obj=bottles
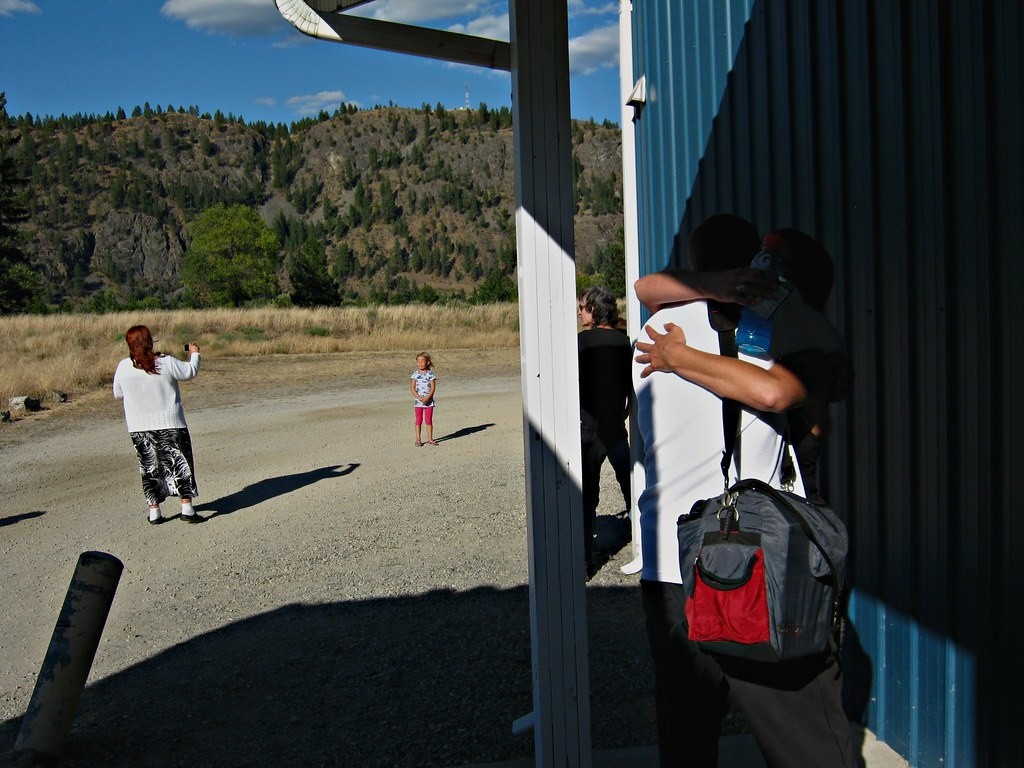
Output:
[734,232,790,357]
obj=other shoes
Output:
[428,441,439,446]
[147,515,166,524]
[180,511,204,523]
[415,440,422,446]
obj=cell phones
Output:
[185,344,189,351]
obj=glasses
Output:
[579,304,585,312]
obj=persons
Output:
[112,324,204,524]
[578,285,632,579]
[410,352,440,446]
[629,214,854,767]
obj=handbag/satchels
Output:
[676,478,850,664]
